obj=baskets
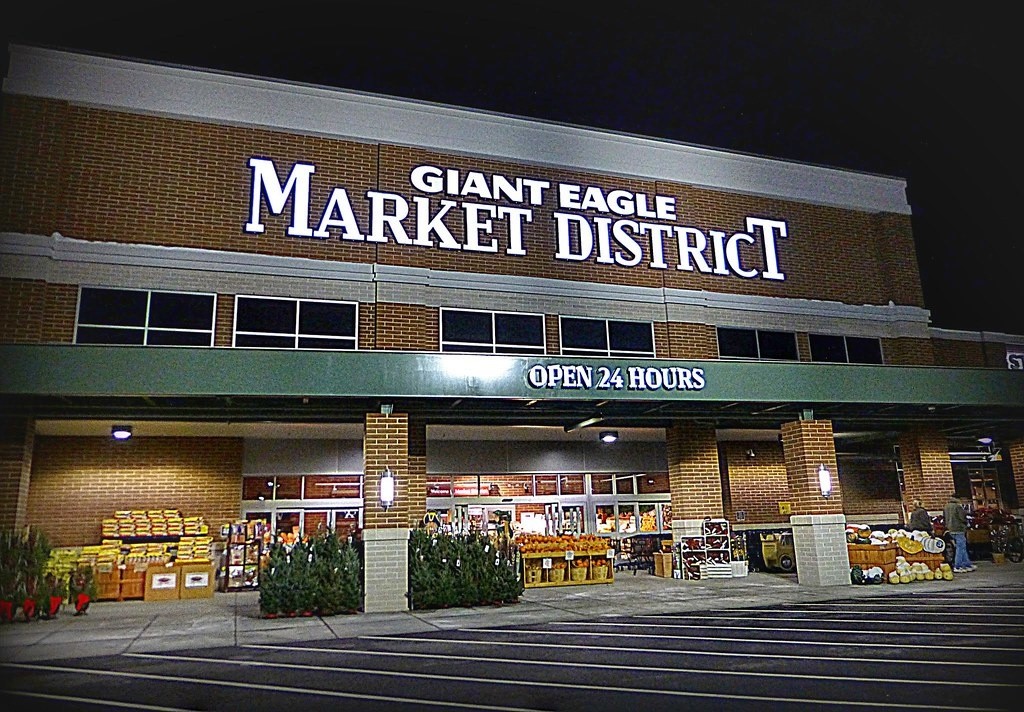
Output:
[549,569,564,582]
[592,561,610,580]
[571,567,587,581]
[526,569,542,582]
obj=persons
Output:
[944,493,978,572]
[908,499,936,538]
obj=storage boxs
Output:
[729,561,749,577]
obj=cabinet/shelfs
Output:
[673,520,732,580]
[71,522,261,604]
[520,548,614,589]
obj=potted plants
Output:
[409,529,525,610]
[258,529,366,619]
[0,527,98,623]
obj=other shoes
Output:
[954,567,967,573]
[966,566,977,571]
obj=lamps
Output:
[818,463,831,499]
[381,468,395,509]
[599,431,618,443]
[111,426,132,439]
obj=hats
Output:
[952,493,960,498]
